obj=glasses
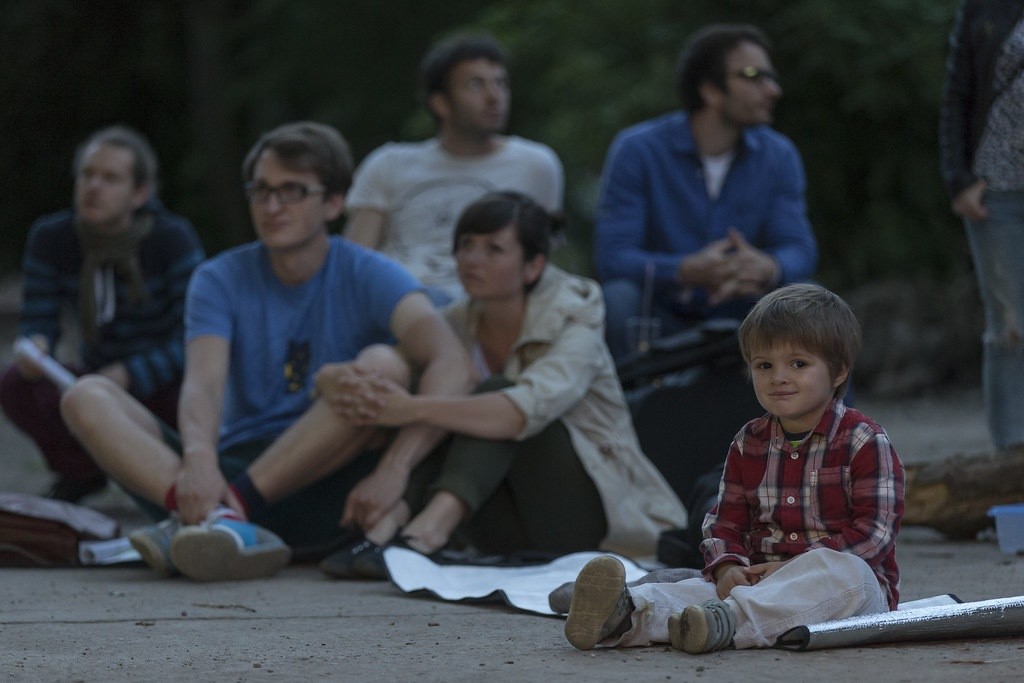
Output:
[727,65,781,85]
[241,181,328,205]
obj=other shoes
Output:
[41,470,109,504]
[667,598,738,654]
[320,535,381,577]
[356,524,450,581]
[563,555,632,651]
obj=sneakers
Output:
[168,515,291,583]
[128,508,186,574]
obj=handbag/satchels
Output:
[616,259,772,478]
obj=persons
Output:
[0,24,820,582]
[564,283,904,654]
[941,0,1024,452]
